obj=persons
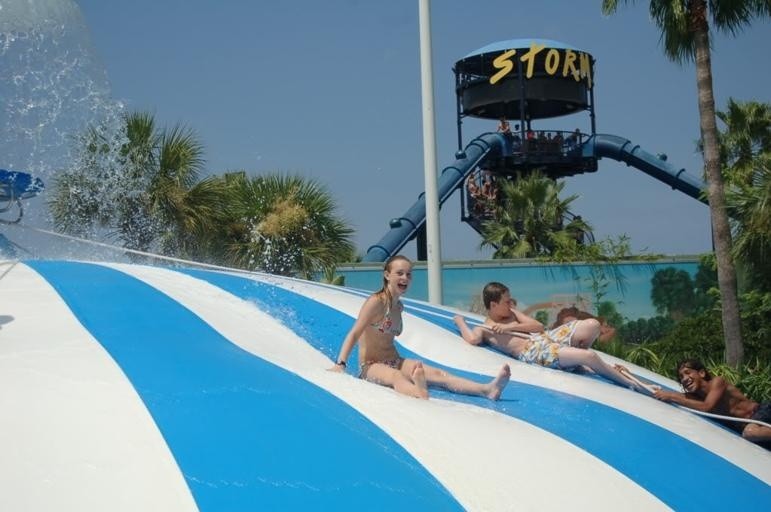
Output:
[571,129,580,144]
[552,132,564,144]
[335,360,348,368]
[495,118,511,139]
[324,254,513,401]
[512,124,522,140]
[453,281,662,400]
[537,131,547,141]
[468,177,500,218]
[651,358,771,443]
